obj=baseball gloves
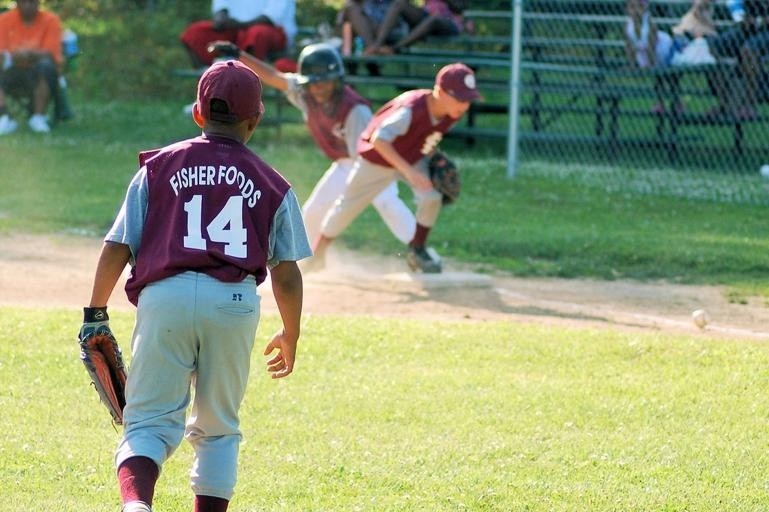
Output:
[79,306,126,424]
[429,154,460,204]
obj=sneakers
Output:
[123,502,150,512]
[0,114,51,134]
[404,241,441,274]
[298,253,327,274]
[651,101,756,119]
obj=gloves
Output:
[208,41,242,60]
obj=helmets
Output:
[297,44,345,85]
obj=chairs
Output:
[0,41,72,126]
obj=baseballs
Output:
[692,310,707,325]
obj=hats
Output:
[197,61,265,124]
[436,64,483,102]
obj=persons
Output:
[207,39,443,271]
[304,62,485,274]
[0,0,64,135]
[181,0,299,115]
[624,0,769,118]
[79,59,313,512]
[339,0,467,77]
